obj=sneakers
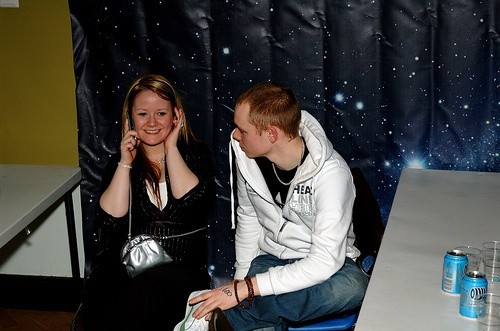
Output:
[173,291,209,331]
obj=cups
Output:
[453,240,500,331]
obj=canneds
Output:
[459,270,488,320]
[441,250,468,296]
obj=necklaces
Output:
[272,137,304,186]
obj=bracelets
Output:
[118,161,133,169]
[233,276,254,310]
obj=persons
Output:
[173,82,365,331]
[72,73,219,331]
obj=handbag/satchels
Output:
[121,235,174,287]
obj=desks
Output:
[353,168,500,331]
[0,163,81,312]
